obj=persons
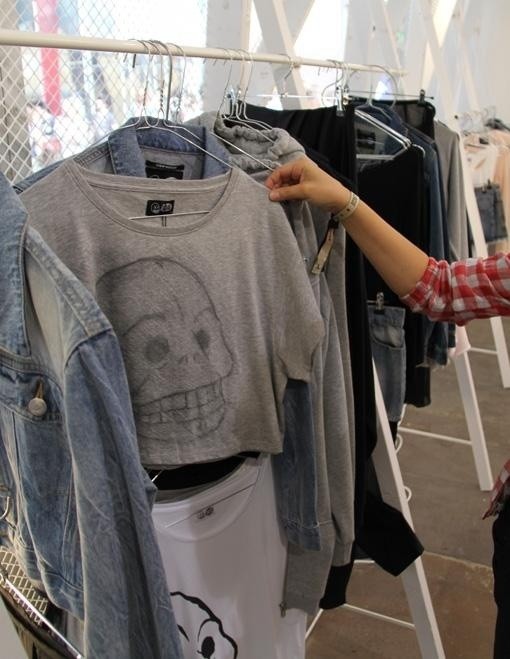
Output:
[265,153,510,658]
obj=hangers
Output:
[9,38,304,225]
[243,51,435,163]
[458,103,510,158]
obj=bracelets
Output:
[332,191,360,221]
